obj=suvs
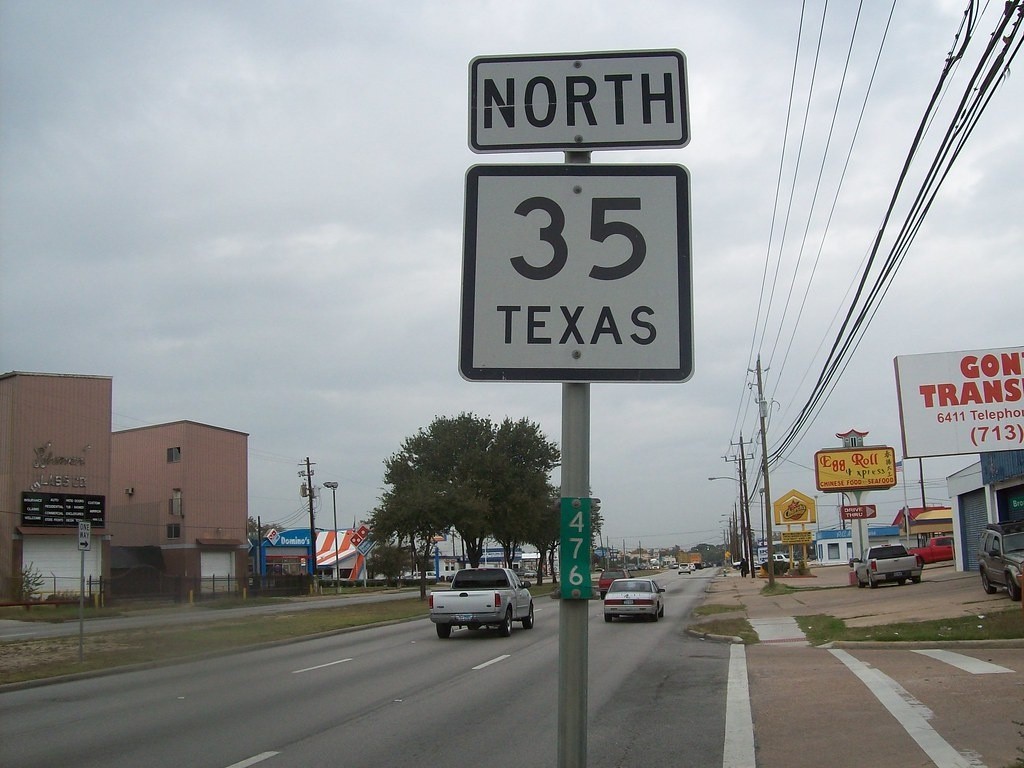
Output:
[977,519,1024,601]
[598,567,635,600]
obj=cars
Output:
[732,551,801,571]
[668,562,679,569]
[677,562,691,574]
[603,578,666,621]
[689,563,696,571]
[694,562,713,569]
[524,569,538,578]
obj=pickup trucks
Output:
[852,544,925,590]
[906,536,955,568]
[428,567,534,639]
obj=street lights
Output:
[708,476,756,578]
[323,481,341,594]
